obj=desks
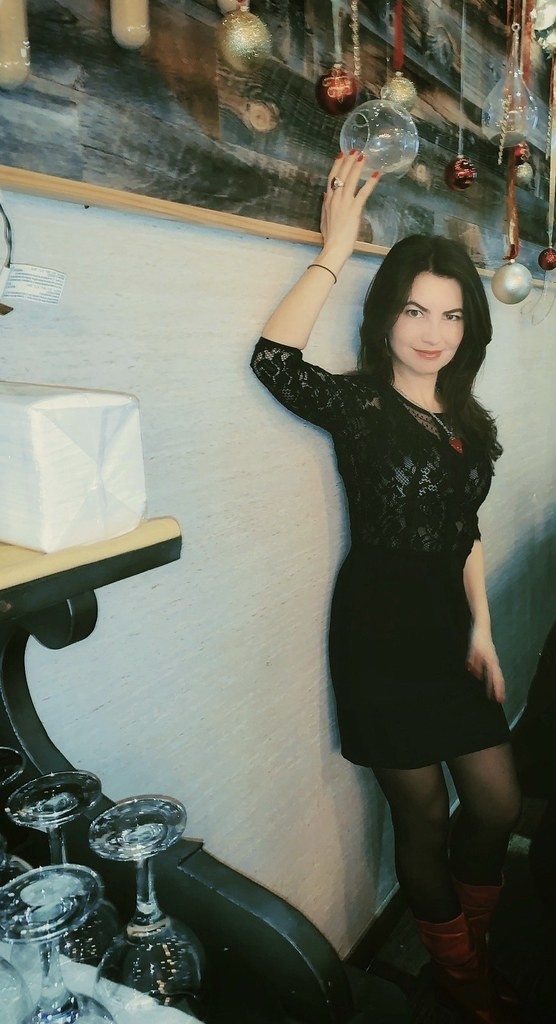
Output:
[0,517,357,1024]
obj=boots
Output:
[450,871,525,1008]
[414,912,527,1024]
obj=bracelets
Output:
[307,264,337,284]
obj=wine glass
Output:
[0,745,208,1023]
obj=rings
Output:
[330,177,344,189]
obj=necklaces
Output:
[394,384,463,453]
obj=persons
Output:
[250,151,520,1024]
